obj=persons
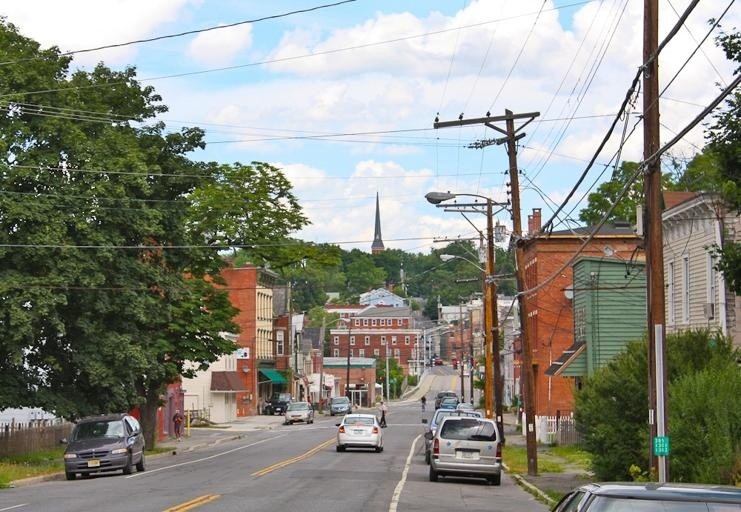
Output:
[173,409,183,441]
[421,396,426,412]
[468,357,474,369]
[379,401,387,427]
[318,397,324,411]
[308,395,312,405]
[452,360,457,370]
[300,385,305,402]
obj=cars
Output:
[61,413,147,479]
[331,397,351,416]
[336,414,384,453]
[286,402,314,424]
[425,408,502,485]
[548,480,741,512]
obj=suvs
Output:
[267,392,292,414]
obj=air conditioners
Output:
[703,303,714,319]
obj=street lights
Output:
[425,191,508,438]
[319,317,351,413]
